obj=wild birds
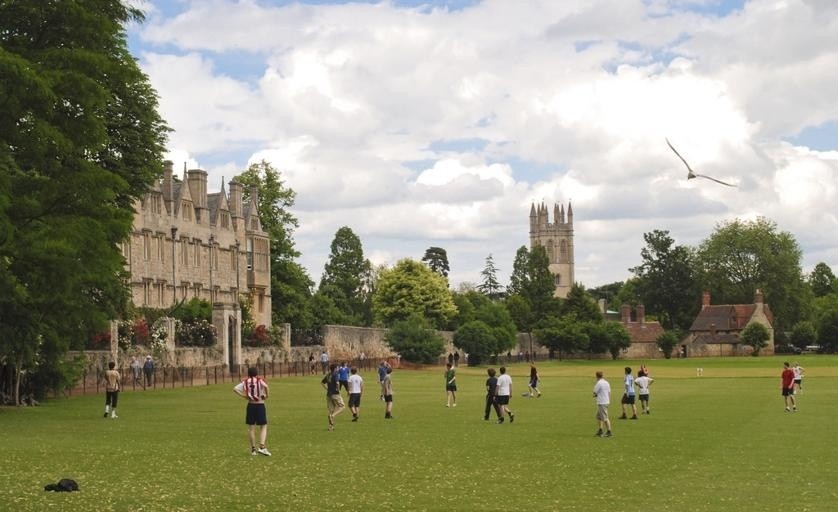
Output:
[663,135,739,190]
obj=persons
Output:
[483,348,541,423]
[792,362,806,395]
[309,349,392,431]
[618,367,637,419]
[780,362,796,411]
[104,362,120,419]
[143,355,155,386]
[593,371,612,437]
[635,371,655,414]
[233,367,271,456]
[129,357,142,390]
[444,347,460,408]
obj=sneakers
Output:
[530,392,542,399]
[618,409,650,420]
[793,391,797,395]
[785,404,797,412]
[251,448,257,456]
[103,410,109,417]
[351,413,359,422]
[110,416,118,419]
[480,412,514,424]
[257,447,272,456]
[385,415,392,419]
[592,431,612,437]
[327,414,335,432]
[446,402,456,407]
